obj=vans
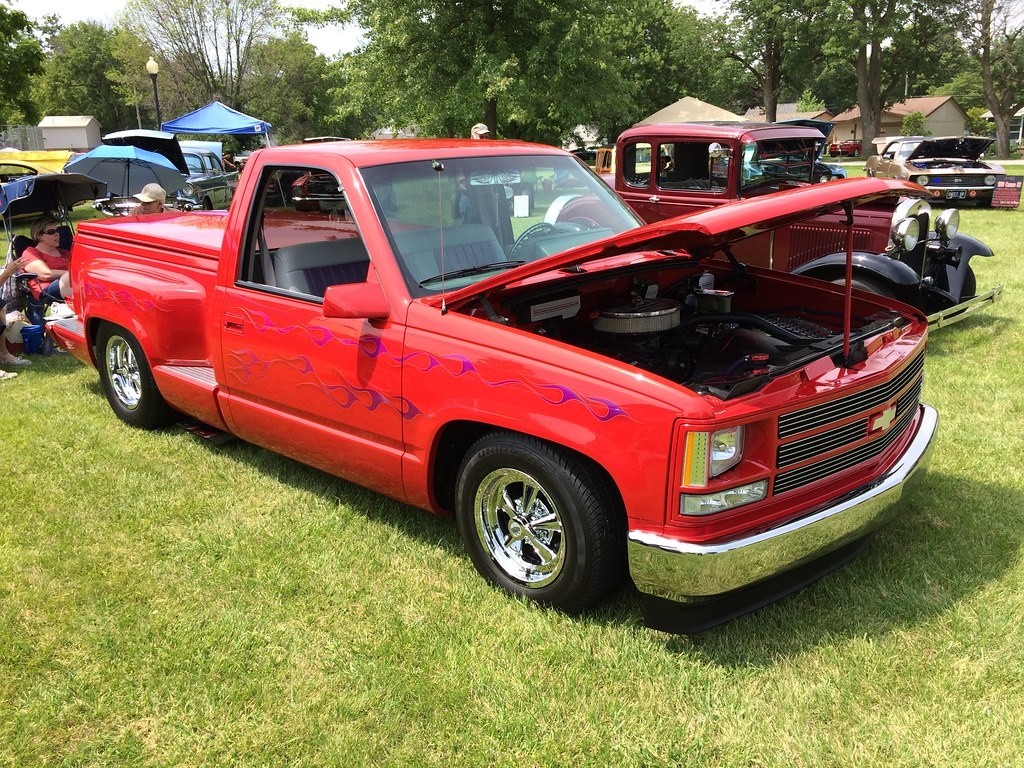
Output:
[595,140,673,174]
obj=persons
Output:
[128,182,182,215]
[221,150,236,172]
[18,216,73,302]
[0,256,32,380]
[456,123,515,248]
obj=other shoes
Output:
[0,370,18,379]
[1,356,32,365]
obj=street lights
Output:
[146,56,161,130]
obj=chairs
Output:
[14,226,75,333]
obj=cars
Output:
[100,130,238,212]
[829,139,862,156]
[47,138,939,611]
[862,133,1005,205]
[291,135,350,212]
[544,125,1003,332]
[711,119,847,191]
[0,160,88,222]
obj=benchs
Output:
[275,222,508,300]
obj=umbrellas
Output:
[63,145,187,198]
[628,94,752,127]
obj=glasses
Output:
[480,136,489,139]
[43,228,60,235]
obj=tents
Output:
[160,101,286,209]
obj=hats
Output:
[133,183,166,203]
[471,123,490,135]
